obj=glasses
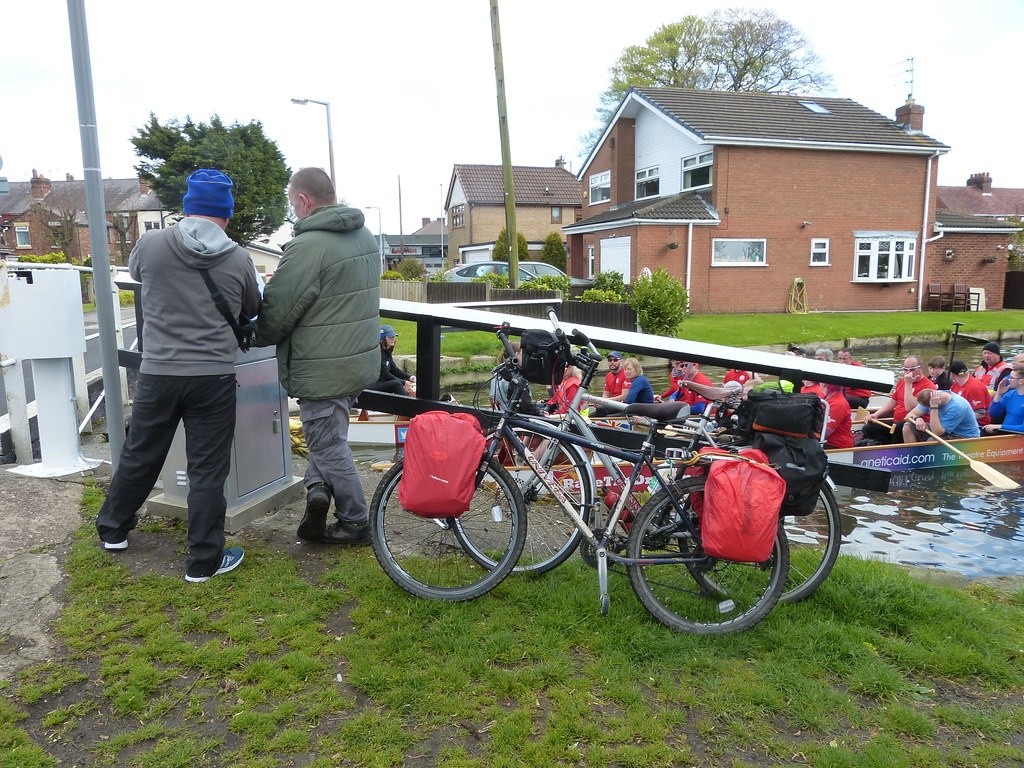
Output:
[608,359,620,362]
[1008,377,1022,382]
[680,364,688,368]
[902,366,921,372]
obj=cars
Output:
[483,260,596,302]
[441,261,541,286]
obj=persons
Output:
[653,359,714,415]
[490,341,543,415]
[1002,353,1024,396]
[980,365,1024,437]
[781,346,871,450]
[95,169,262,582]
[947,361,991,428]
[544,365,589,416]
[589,350,631,418]
[903,388,980,444]
[715,368,764,446]
[249,169,381,548]
[623,358,654,403]
[972,342,1013,400]
[373,324,416,397]
[926,356,949,391]
[862,356,936,442]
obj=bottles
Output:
[704,420,719,431]
[647,458,677,494]
[602,485,636,531]
[580,400,590,418]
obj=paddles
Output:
[853,406,895,433]
[904,416,1020,489]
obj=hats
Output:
[949,361,968,375]
[182,169,234,218]
[380,325,399,340]
[607,351,622,360]
[789,346,806,354]
[982,342,1000,355]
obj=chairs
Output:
[927,283,955,310]
[952,283,981,312]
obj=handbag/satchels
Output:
[519,329,565,386]
[690,446,769,514]
[753,432,829,516]
[237,312,259,353]
[740,389,823,441]
[701,458,786,563]
[398,411,486,518]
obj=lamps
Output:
[800,220,811,230]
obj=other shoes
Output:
[319,510,373,545]
[297,486,331,540]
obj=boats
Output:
[284,385,1023,495]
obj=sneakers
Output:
[185,547,244,582]
[102,535,129,549]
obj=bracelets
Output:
[930,407,938,410]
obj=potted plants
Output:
[984,256,998,263]
[667,241,680,249]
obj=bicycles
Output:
[364,319,792,636]
[444,302,845,609]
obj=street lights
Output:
[365,205,384,274]
[290,97,336,194]
